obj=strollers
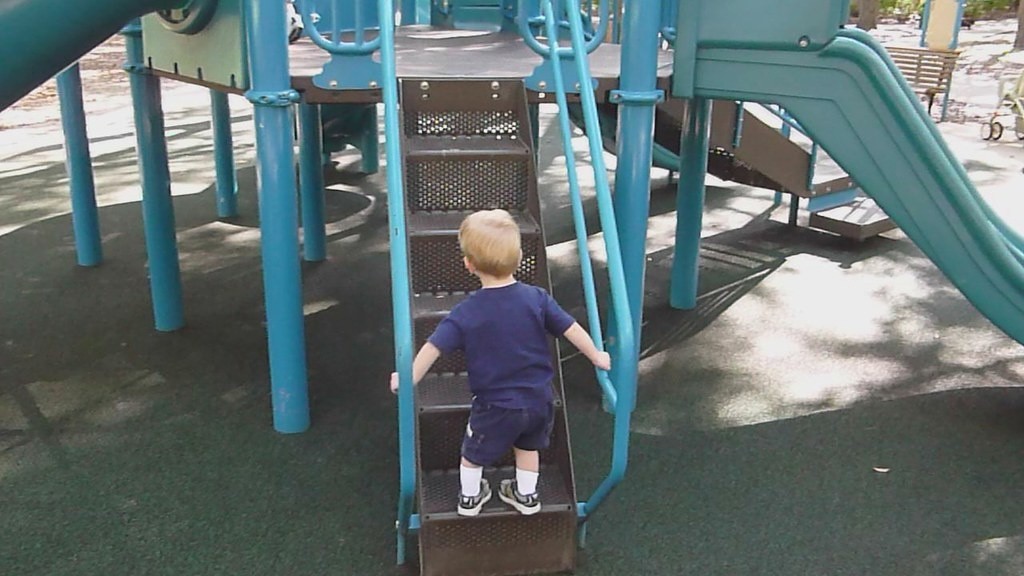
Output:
[979,51,1024,142]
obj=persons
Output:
[390,209,611,517]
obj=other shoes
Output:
[288,14,304,43]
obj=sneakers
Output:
[496,478,542,516]
[456,479,492,516]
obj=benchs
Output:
[883,46,960,122]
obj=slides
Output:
[563,97,682,169]
[800,25,1024,347]
